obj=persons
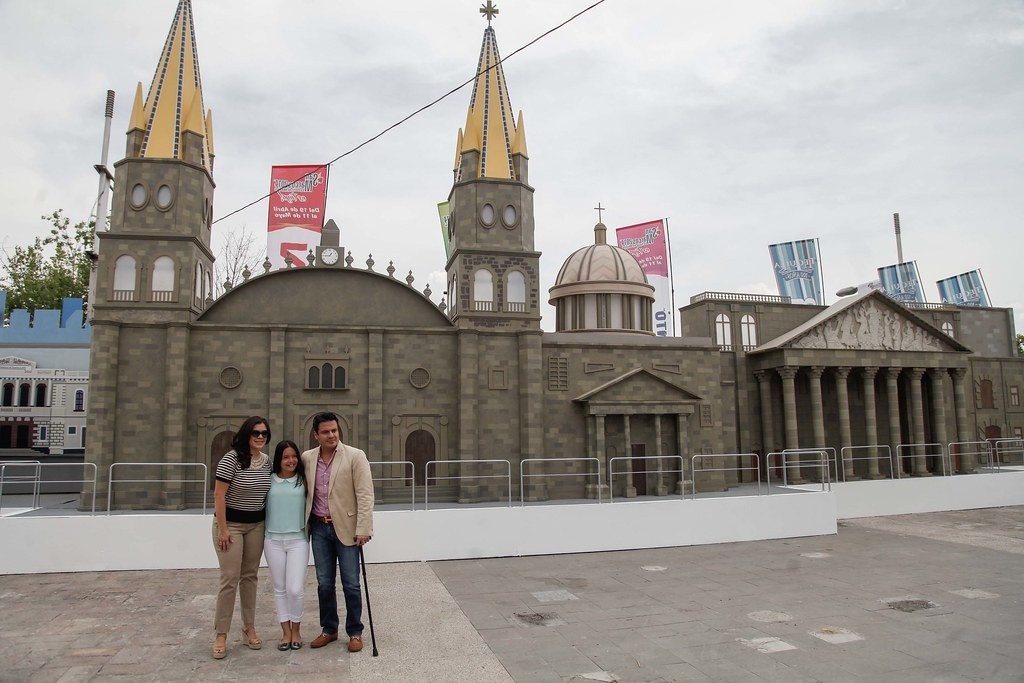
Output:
[794,299,942,351]
[264,440,310,649]
[211,416,273,659]
[300,413,375,652]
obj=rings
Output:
[361,541,365,544]
[219,544,222,545]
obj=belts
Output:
[313,515,332,523]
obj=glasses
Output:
[251,430,270,439]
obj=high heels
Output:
[241,625,261,649]
[213,633,227,658]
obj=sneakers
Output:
[311,633,338,647]
[348,636,362,651]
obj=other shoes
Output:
[278,639,291,650]
[291,641,302,649]
